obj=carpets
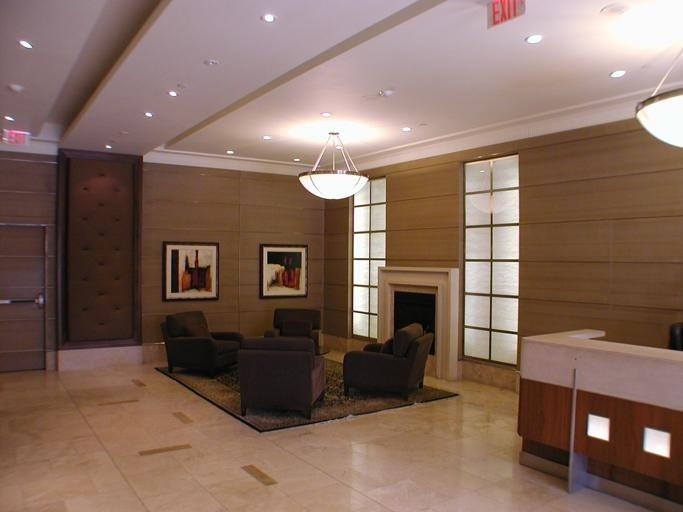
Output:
[154,353,459,433]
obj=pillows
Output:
[192,327,215,344]
[282,327,310,336]
[379,337,392,353]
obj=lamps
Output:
[297,131,369,200]
[631,48,683,148]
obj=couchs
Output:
[159,310,245,381]
[263,307,323,354]
[342,323,436,399]
[237,336,324,420]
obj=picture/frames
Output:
[160,240,220,301]
[258,243,308,299]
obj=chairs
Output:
[667,323,682,351]
